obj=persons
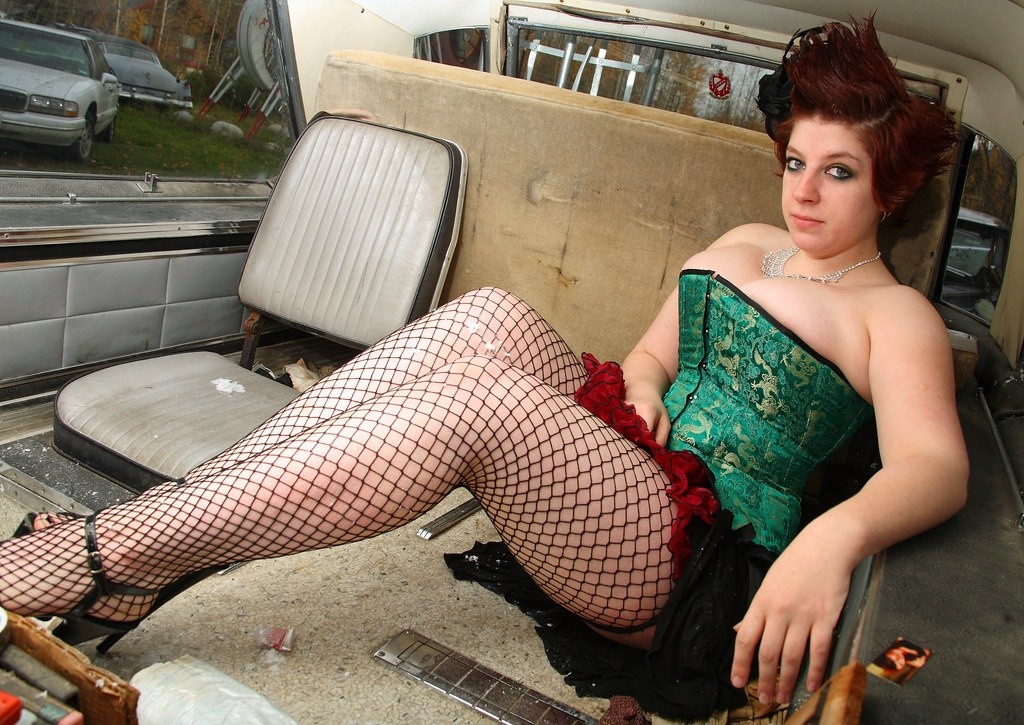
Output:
[0,15,968,704]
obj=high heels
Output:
[0,508,159,647]
[13,478,229,621]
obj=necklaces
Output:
[761,246,881,284]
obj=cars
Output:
[1,20,194,163]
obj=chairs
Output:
[47,109,479,494]
